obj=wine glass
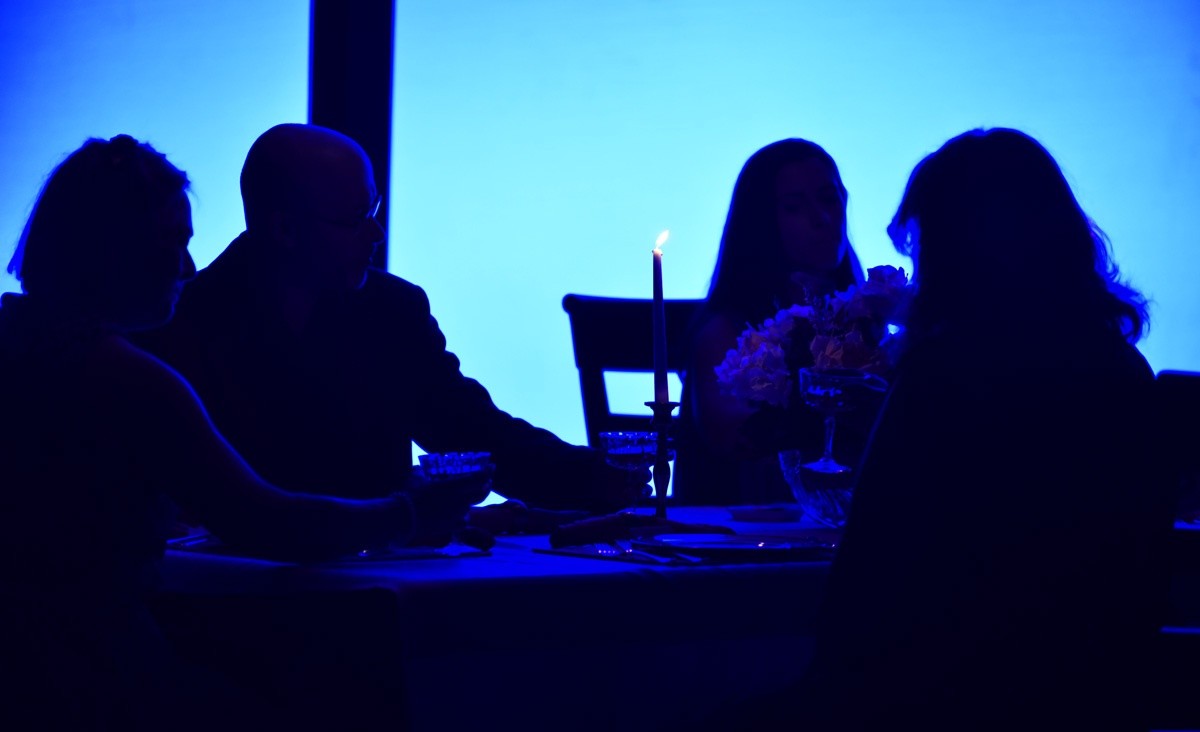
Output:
[418,453,496,557]
[598,432,662,516]
[800,369,853,473]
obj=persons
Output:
[170,124,652,511]
[672,138,867,507]
[0,134,495,732]
[802,127,1170,732]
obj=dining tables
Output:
[155,502,847,732]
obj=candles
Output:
[652,249,670,402]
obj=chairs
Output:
[563,292,705,500]
